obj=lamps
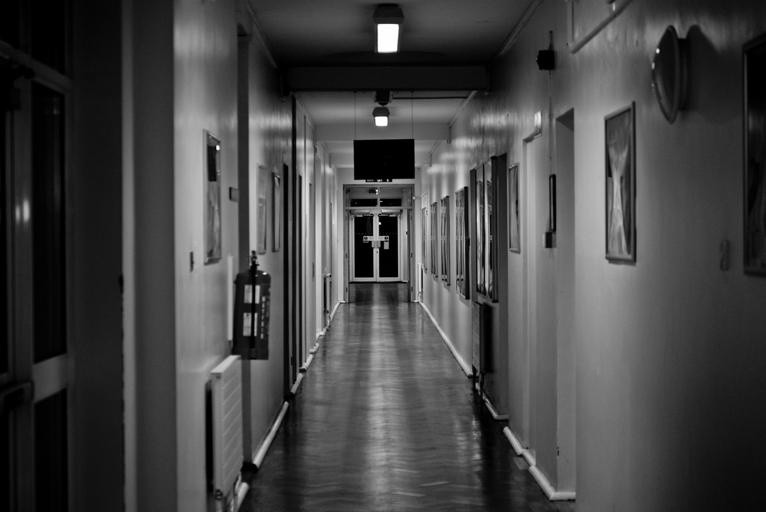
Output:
[372,2,404,129]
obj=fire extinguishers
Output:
[233,250,271,360]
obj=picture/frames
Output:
[602,99,637,268]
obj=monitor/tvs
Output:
[353,139,416,179]
